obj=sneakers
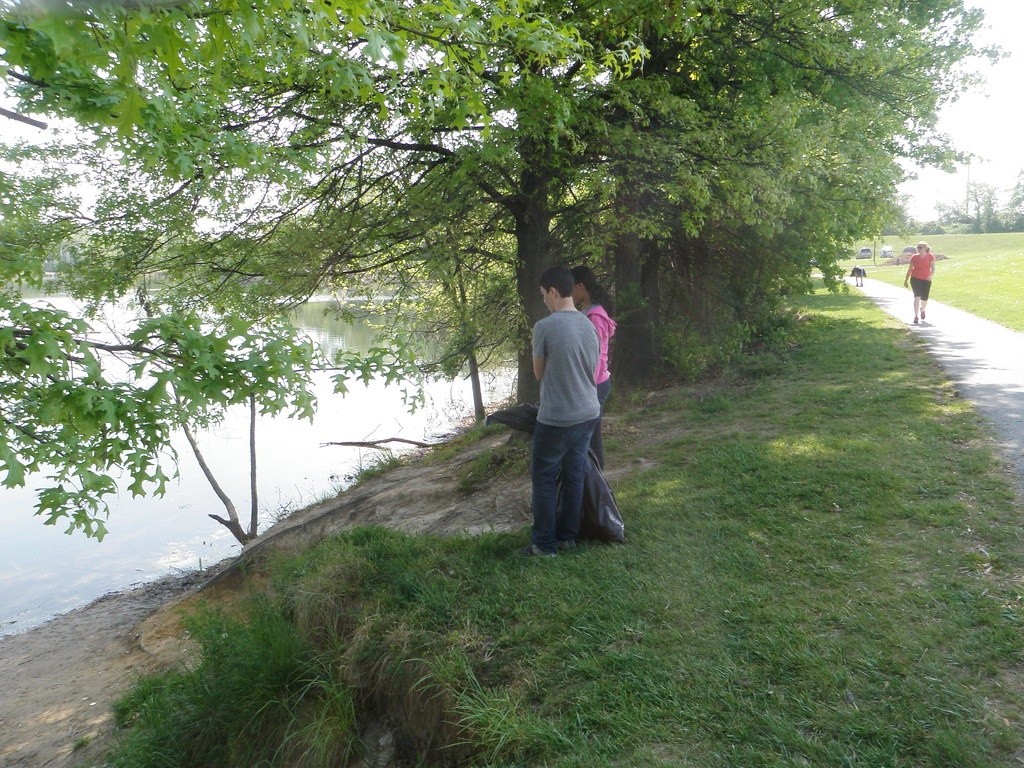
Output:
[514,545,557,558]
[556,538,575,550]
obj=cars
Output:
[902,246,919,254]
[878,247,896,258]
[855,248,873,259]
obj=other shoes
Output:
[920,308,925,319]
[913,317,918,323]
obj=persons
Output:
[904,242,936,324]
[571,264,617,471]
[530,268,603,559]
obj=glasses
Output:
[917,247,926,249]
